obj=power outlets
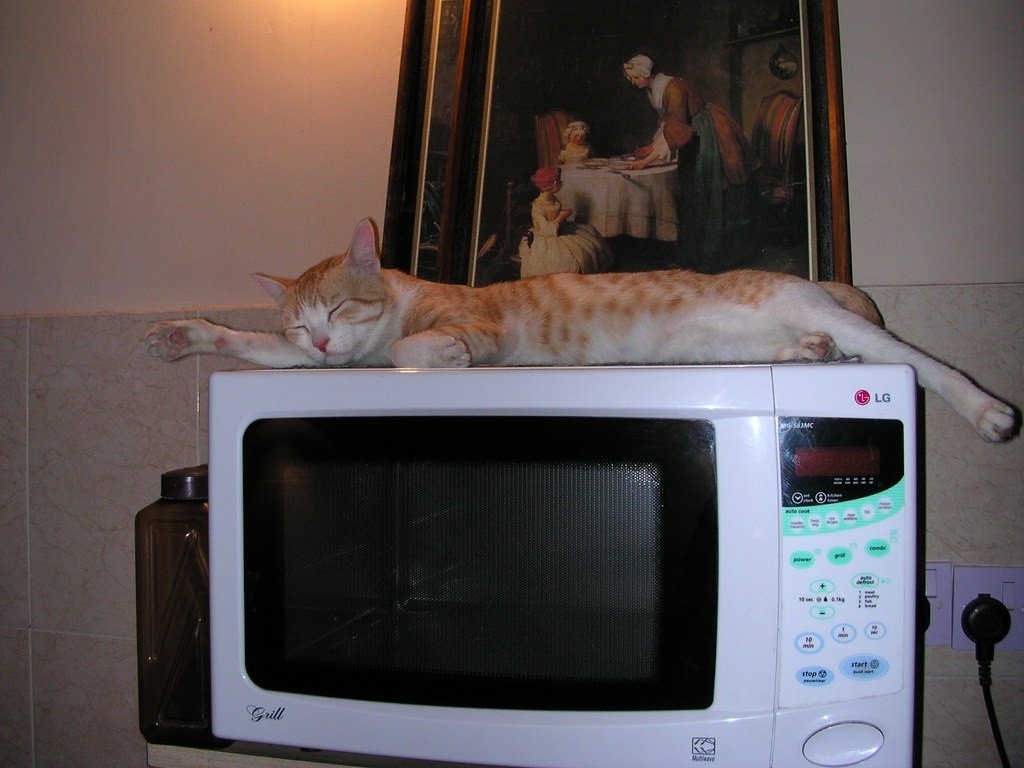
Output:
[953,565,1024,655]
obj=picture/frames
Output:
[380,0,853,285]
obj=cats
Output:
[145,218,1016,443]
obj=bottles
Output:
[134,469,232,749]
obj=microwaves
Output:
[207,364,916,768]
